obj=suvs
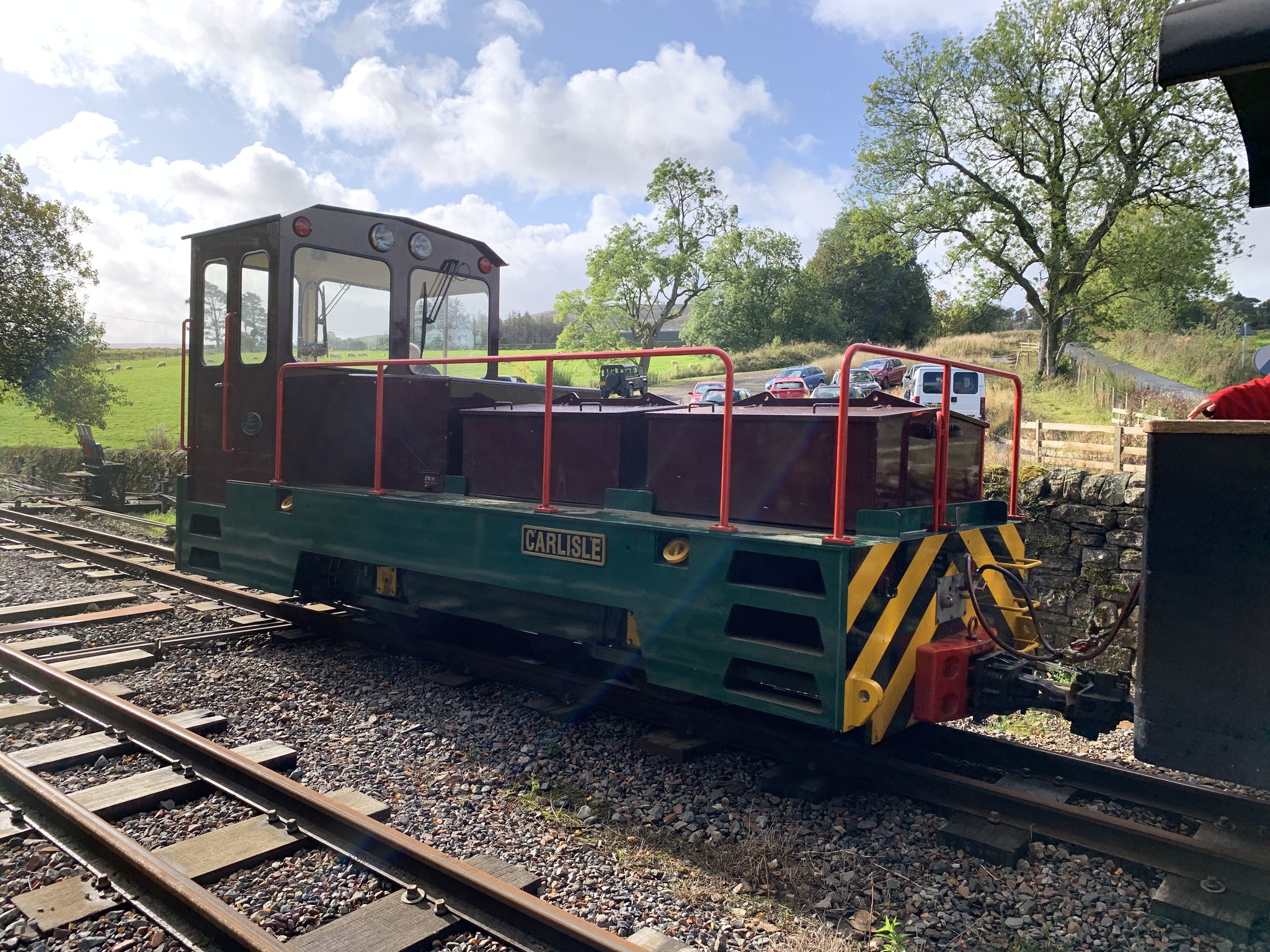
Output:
[768,376,810,400]
[809,384,866,399]
[829,366,883,398]
[764,365,827,392]
[599,364,648,399]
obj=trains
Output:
[172,1,1269,792]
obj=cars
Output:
[901,362,945,391]
[697,389,753,408]
[859,358,905,389]
[687,381,726,404]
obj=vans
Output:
[904,367,987,422]
